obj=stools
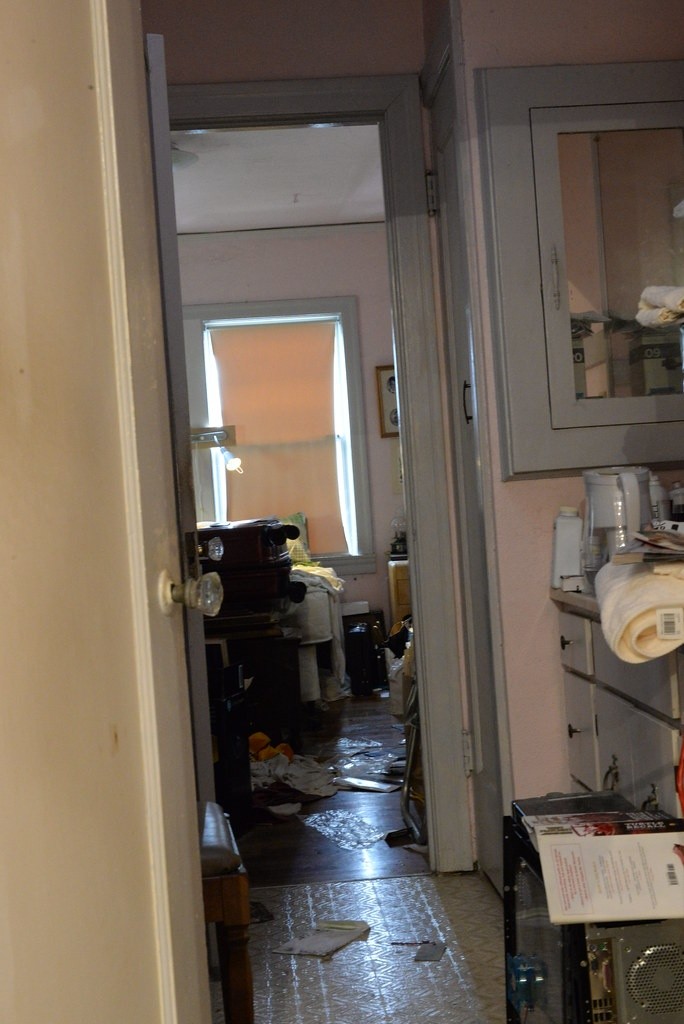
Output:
[196,799,256,1024]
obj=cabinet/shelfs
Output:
[556,602,684,818]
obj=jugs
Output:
[581,466,653,587]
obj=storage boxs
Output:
[571,336,616,399]
[627,333,683,397]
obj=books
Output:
[522,810,684,925]
[611,520,684,564]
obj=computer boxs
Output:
[501,813,684,1024]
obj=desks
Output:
[386,559,412,625]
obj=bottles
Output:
[649,475,684,522]
[550,506,583,589]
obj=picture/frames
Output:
[374,365,400,438]
